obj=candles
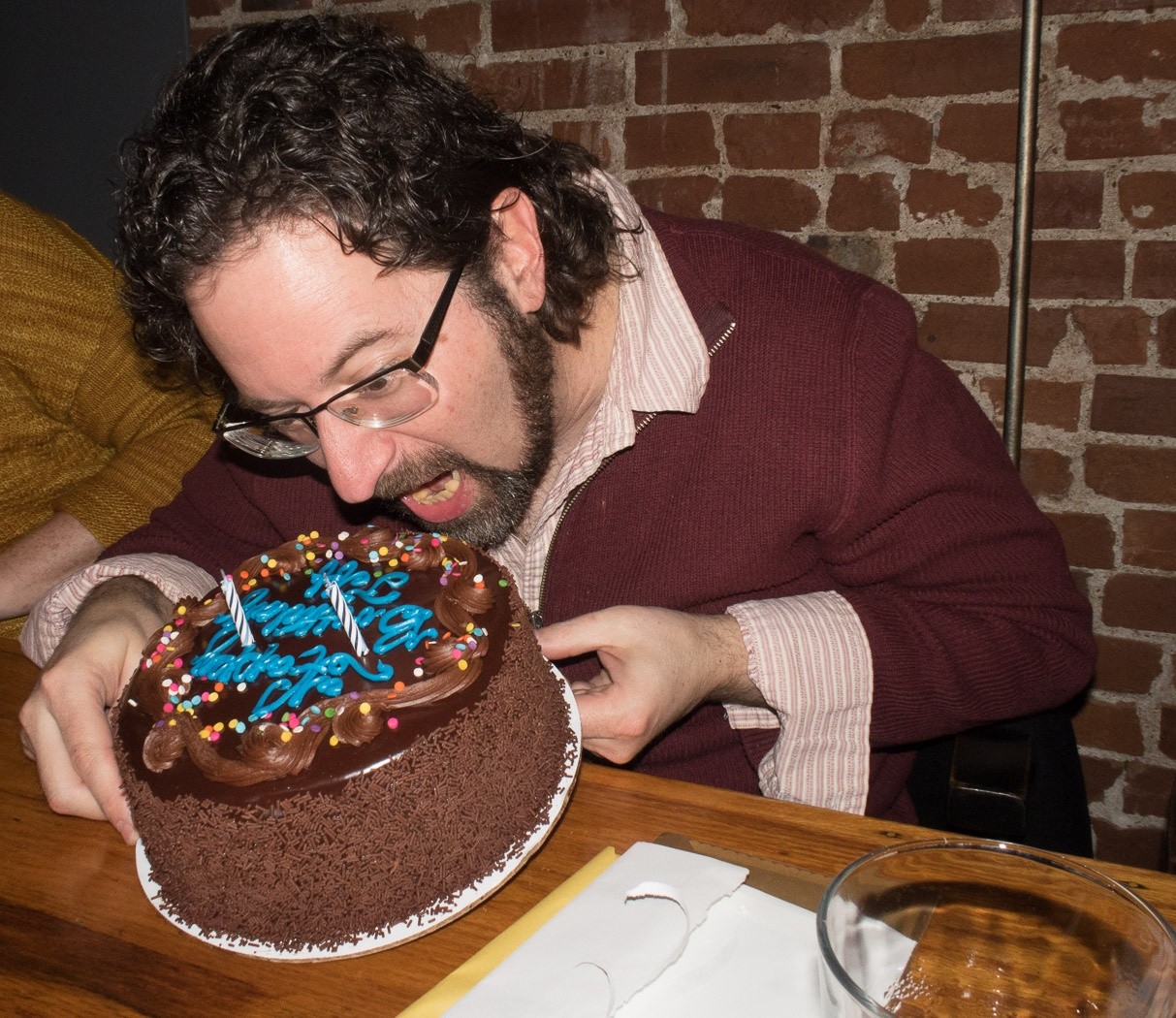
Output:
[321,572,369,656]
[217,568,255,646]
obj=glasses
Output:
[208,224,488,460]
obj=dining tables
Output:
[0,642,1176,1018]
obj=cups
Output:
[816,838,1176,1018]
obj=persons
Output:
[0,189,226,623]
[20,11,1099,849]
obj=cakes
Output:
[108,529,573,950]
